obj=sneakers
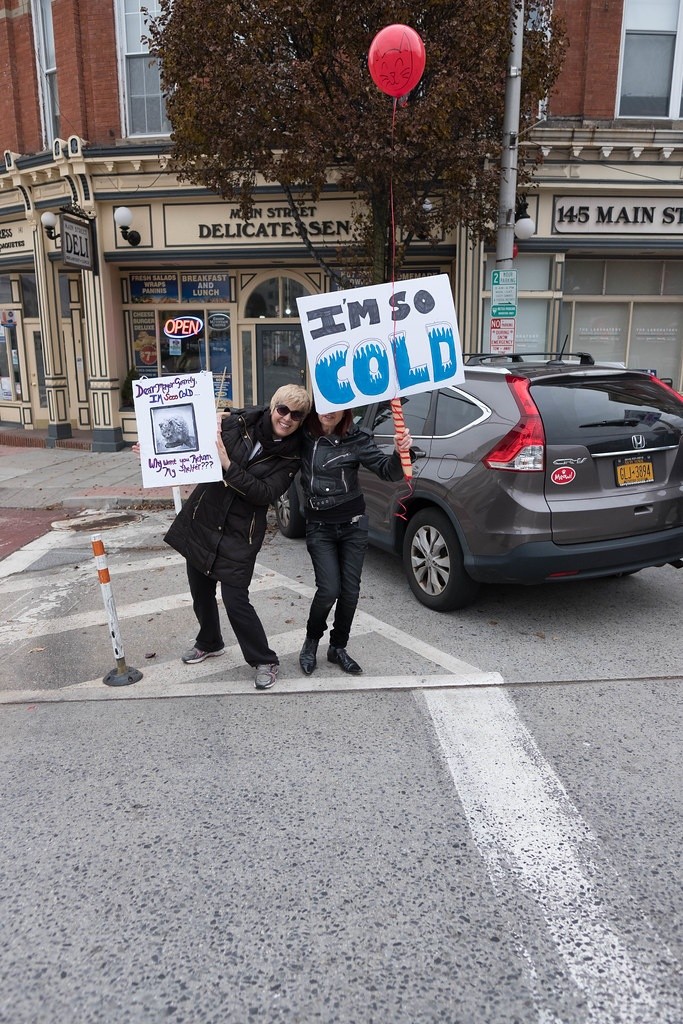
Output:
[180,645,225,664]
[254,663,279,689]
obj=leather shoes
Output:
[298,637,319,676]
[326,645,364,674]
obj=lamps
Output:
[114,207,141,246]
[515,198,536,240]
[41,211,61,240]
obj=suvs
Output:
[257,349,682,611]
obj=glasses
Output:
[275,404,303,421]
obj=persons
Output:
[131,384,311,690]
[216,388,413,674]
